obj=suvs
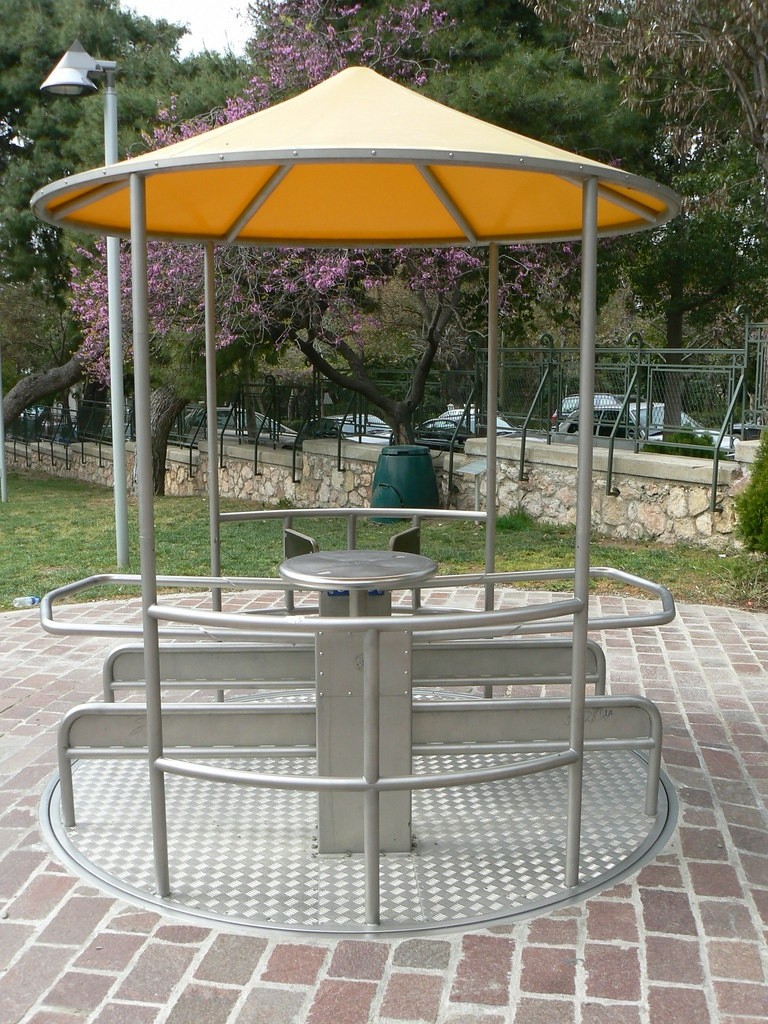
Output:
[550,402,740,461]
[551,392,646,427]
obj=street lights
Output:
[37,38,132,574]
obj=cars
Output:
[22,404,60,427]
[300,413,396,445]
[181,405,298,451]
[426,409,521,438]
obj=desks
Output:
[278,547,438,617]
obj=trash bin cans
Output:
[369,444,439,524]
